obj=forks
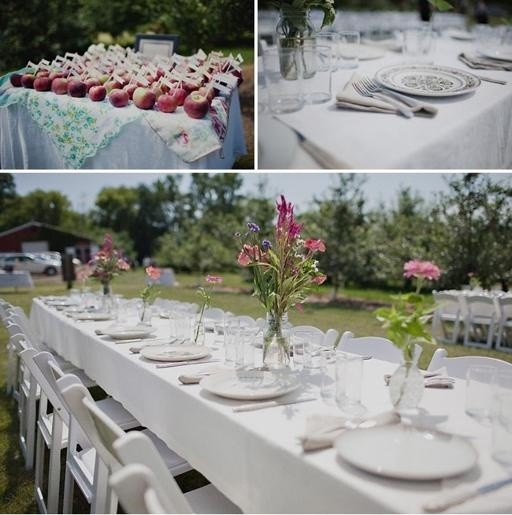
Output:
[354,73,434,120]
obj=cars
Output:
[0,251,82,276]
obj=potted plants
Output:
[270,1,337,80]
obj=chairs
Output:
[0,291,512,514]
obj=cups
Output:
[360,12,510,63]
[87,289,364,409]
[452,366,512,471]
[258,27,361,115]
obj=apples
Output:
[10,55,243,119]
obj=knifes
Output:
[421,476,512,514]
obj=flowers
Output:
[83,233,130,295]
[234,195,328,313]
[139,266,162,304]
[192,276,223,322]
[373,260,441,348]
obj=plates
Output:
[335,422,480,483]
[46,293,297,402]
[377,62,480,99]
